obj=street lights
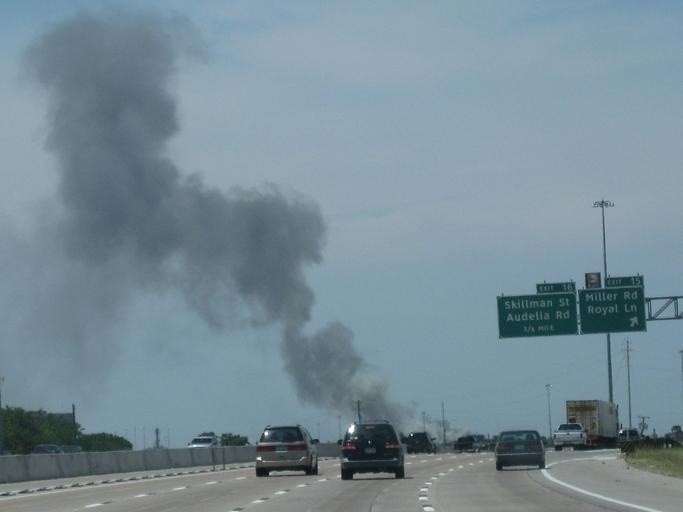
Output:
[544,383,553,441]
[591,198,616,403]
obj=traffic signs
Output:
[496,272,646,340]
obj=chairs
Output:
[504,434,535,442]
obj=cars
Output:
[254,424,320,478]
[406,431,439,453]
[494,429,546,471]
[187,436,218,449]
[337,422,405,481]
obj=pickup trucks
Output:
[552,421,588,451]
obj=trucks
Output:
[565,398,619,449]
